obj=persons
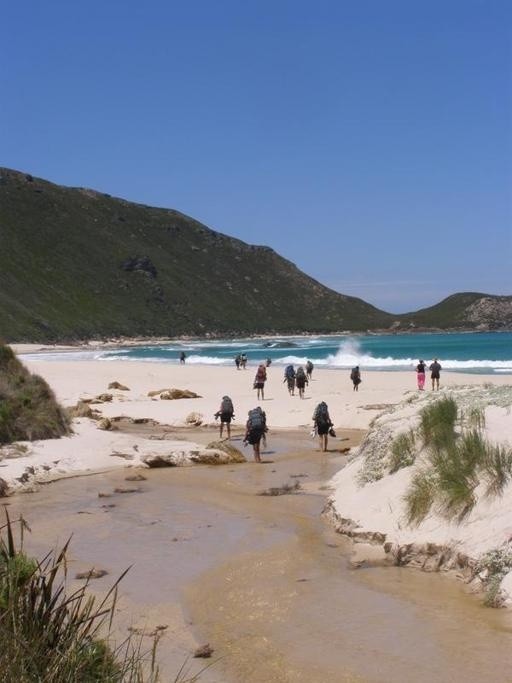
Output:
[253,364,267,400]
[213,395,235,439]
[180,351,186,365]
[415,360,426,391]
[295,367,308,399]
[351,366,360,391]
[283,365,295,396]
[305,361,313,379]
[240,353,247,369]
[234,352,241,369]
[243,406,269,462]
[429,358,441,391]
[313,401,333,452]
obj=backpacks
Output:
[351,369,359,381]
[316,403,330,431]
[258,367,265,382]
[249,409,265,433]
[285,363,313,384]
[222,399,233,417]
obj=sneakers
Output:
[330,429,336,437]
